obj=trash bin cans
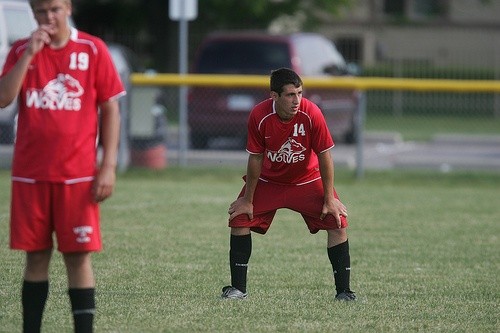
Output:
[132,134,167,170]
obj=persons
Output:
[221,68,356,301]
[0,0,127,333]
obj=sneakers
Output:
[220,287,247,300]
[334,289,359,303]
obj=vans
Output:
[186,33,367,151]
